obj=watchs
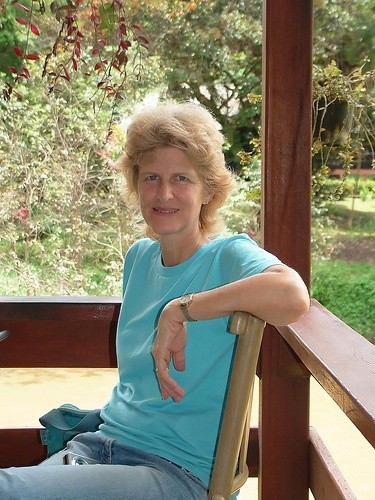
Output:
[179,294,197,322]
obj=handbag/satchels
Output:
[39,403,104,458]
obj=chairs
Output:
[207,310,267,500]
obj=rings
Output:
[155,368,158,372]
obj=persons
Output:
[0,103,309,500]
